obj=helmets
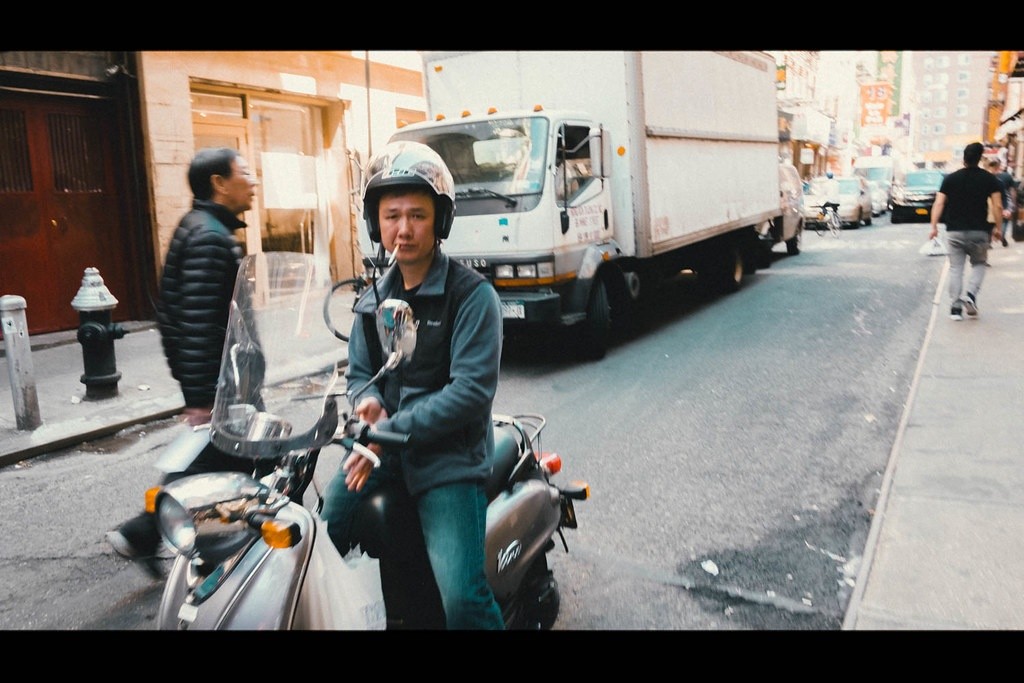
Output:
[364,150,454,242]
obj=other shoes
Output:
[105,531,168,582]
[1002,237,1008,246]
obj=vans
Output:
[853,156,897,205]
[777,163,806,255]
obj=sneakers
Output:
[951,307,963,319]
[963,294,978,315]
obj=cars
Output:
[801,176,873,230]
[866,181,888,218]
[891,170,948,225]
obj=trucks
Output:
[343,51,784,363]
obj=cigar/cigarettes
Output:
[388,244,400,265]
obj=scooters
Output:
[144,252,589,631]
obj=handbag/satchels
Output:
[920,237,949,255]
[294,510,387,630]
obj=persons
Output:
[819,172,840,216]
[549,156,579,201]
[105,147,304,583]
[984,157,1017,267]
[309,140,507,630]
[929,142,1002,321]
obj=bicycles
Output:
[321,274,370,345]
[815,206,842,239]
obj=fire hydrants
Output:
[70,266,132,404]
